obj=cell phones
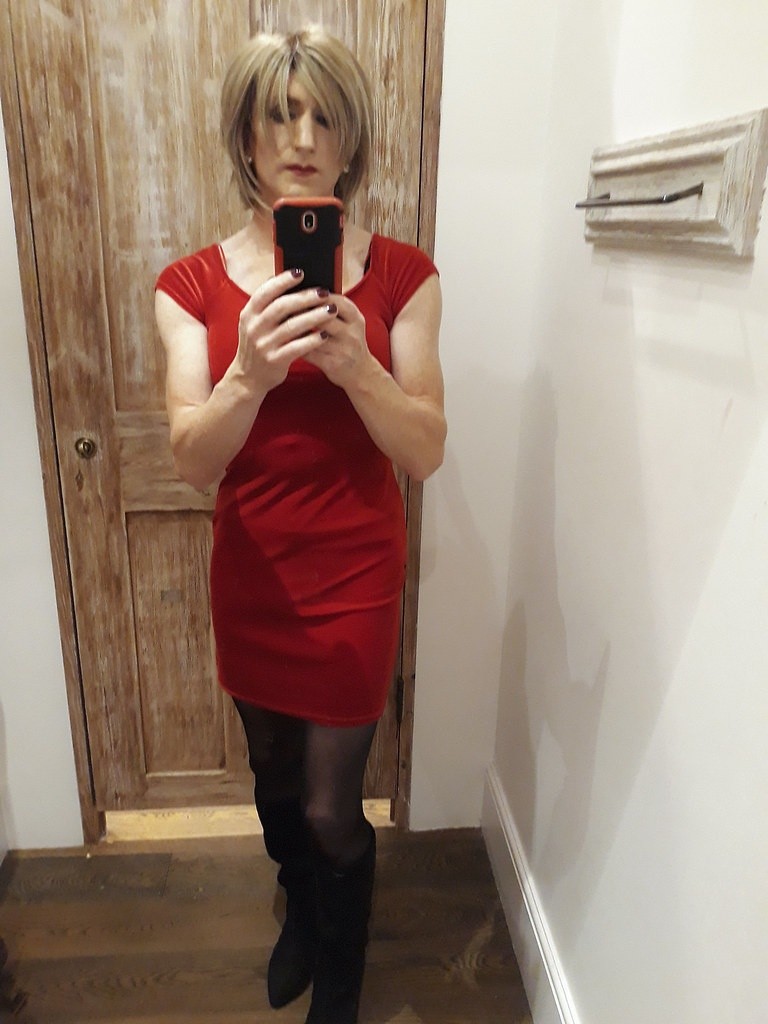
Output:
[272,196,346,323]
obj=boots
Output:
[255,797,322,1008]
[305,820,375,1024]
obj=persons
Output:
[150,21,448,1024]
